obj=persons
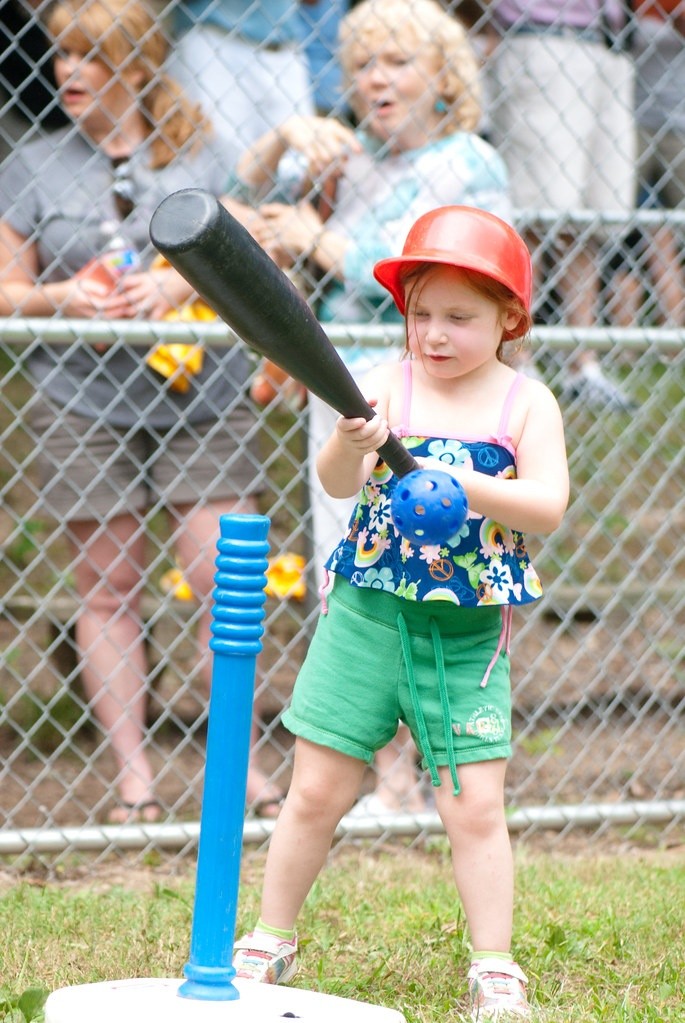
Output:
[234,200,571,1023]
[0,0,285,832]
[237,0,515,825]
[142,0,321,404]
[621,2,685,341]
[477,0,644,422]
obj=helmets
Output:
[372,204,533,343]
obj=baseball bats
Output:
[149,187,423,480]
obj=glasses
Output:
[111,154,136,224]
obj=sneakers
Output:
[231,929,301,985]
[466,957,530,1023]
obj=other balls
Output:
[390,469,469,547]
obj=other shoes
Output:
[107,795,160,823]
[245,792,288,819]
[561,366,641,410]
[339,795,440,830]
